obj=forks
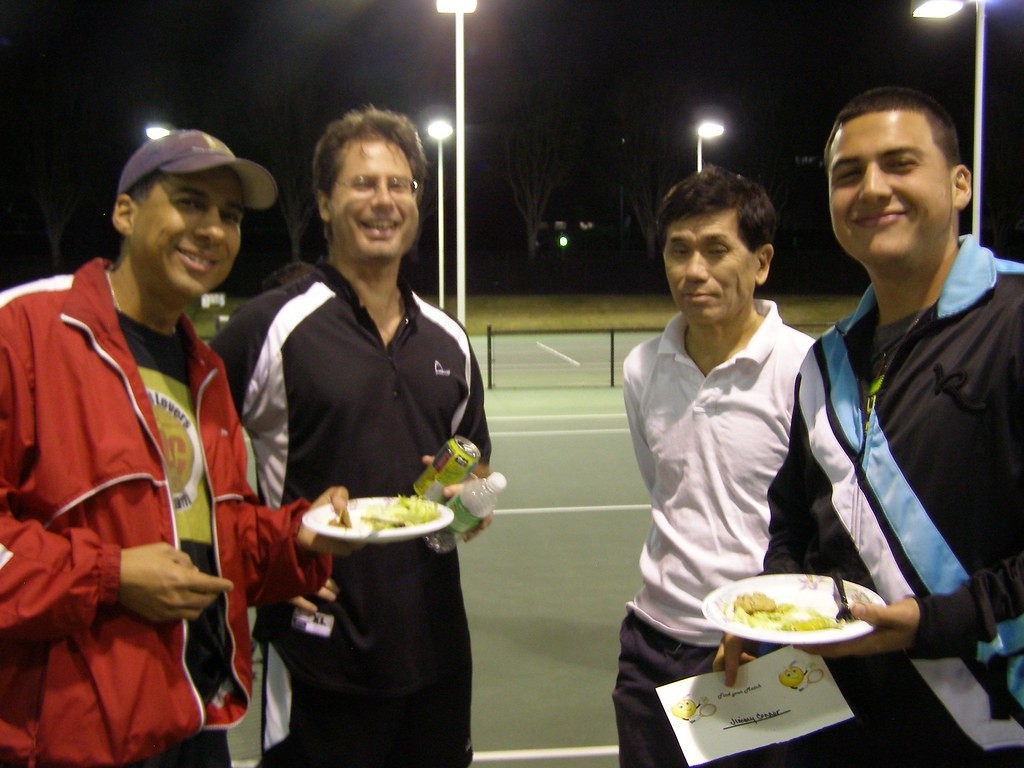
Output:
[831,569,856,623]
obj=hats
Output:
[117,129,277,210]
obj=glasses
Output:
[334,174,422,200]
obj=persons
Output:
[611,165,816,768]
[210,110,494,768]
[712,90,1024,768]
[0,129,349,768]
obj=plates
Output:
[702,574,886,644]
[302,497,454,543]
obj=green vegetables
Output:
[366,493,441,533]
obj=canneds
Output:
[413,434,481,505]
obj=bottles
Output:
[423,473,507,553]
